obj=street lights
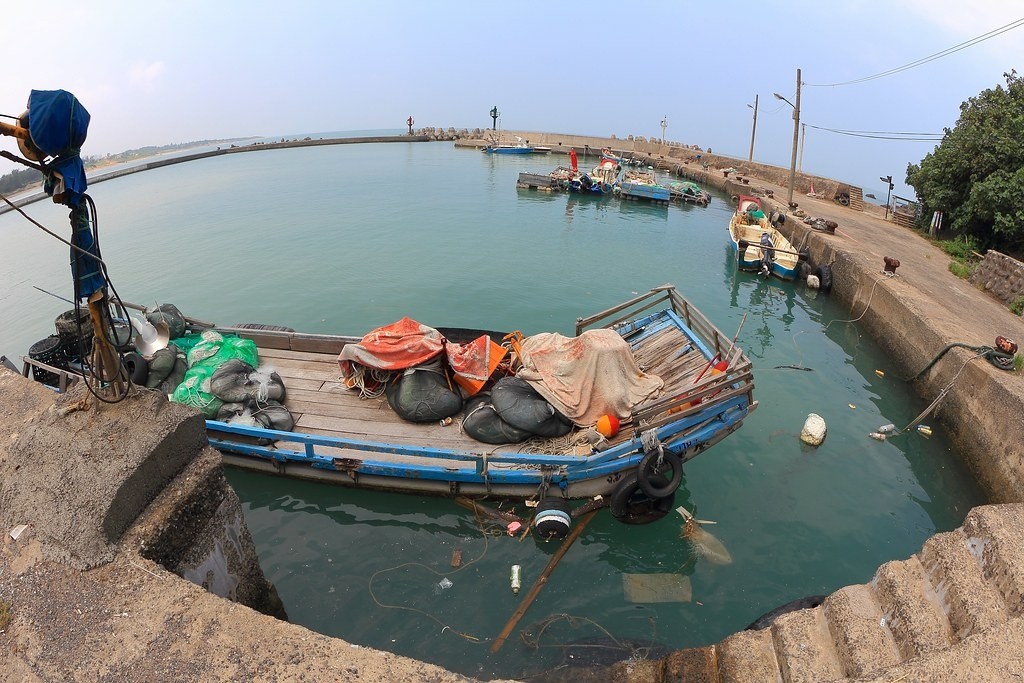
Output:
[772,92,801,201]
[747,103,757,163]
[880,175,892,218]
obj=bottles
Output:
[511,565,521,593]
[918,428,932,435]
[878,424,894,433]
[870,433,885,440]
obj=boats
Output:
[22,294,759,540]
[517,148,713,208]
[728,197,808,284]
[480,138,553,154]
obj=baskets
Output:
[29,337,71,386]
[55,307,94,358]
